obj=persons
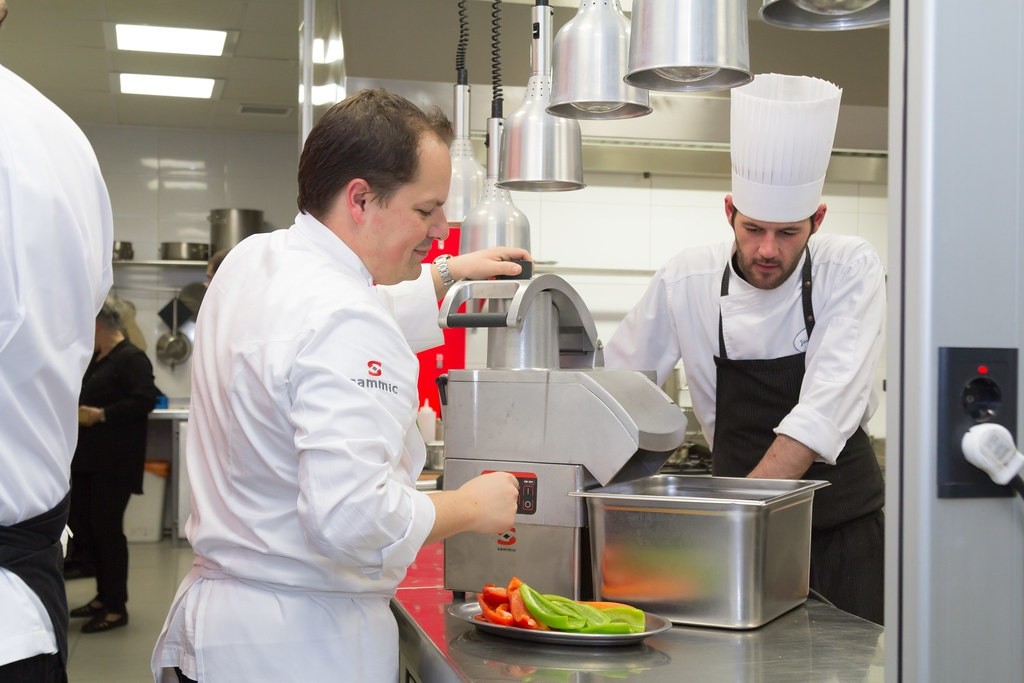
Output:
[70,299,155,631]
[604,73,884,624]
[1,62,112,683]
[152,93,535,683]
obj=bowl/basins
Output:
[112,242,133,260]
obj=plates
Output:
[446,600,673,645]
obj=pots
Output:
[156,298,192,368]
[159,242,209,260]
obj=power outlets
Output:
[940,348,1019,498]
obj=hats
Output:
[730,72,844,222]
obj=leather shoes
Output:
[81,610,127,634]
[70,602,100,618]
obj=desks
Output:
[146,409,189,546]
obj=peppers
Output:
[473,578,645,635]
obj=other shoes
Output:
[63,556,93,580]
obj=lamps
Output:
[445,0,890,256]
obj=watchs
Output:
[432,254,455,288]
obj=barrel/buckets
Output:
[207,208,265,261]
